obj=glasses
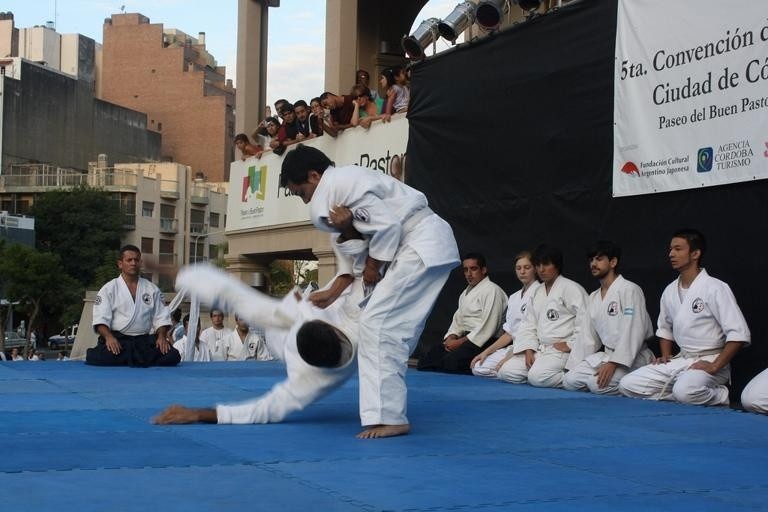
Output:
[353,93,365,100]
[357,75,367,79]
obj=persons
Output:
[309,98,326,138]
[471,254,540,377]
[379,70,396,122]
[86,244,181,367]
[150,205,371,424]
[234,133,263,161]
[281,104,300,141]
[30,329,36,348]
[383,66,409,123]
[28,349,38,360]
[199,310,232,362]
[293,100,312,139]
[172,315,210,361]
[418,253,508,375]
[165,308,181,344]
[740,366,767,414]
[252,117,281,160]
[350,84,377,127]
[617,230,751,406]
[220,312,271,361]
[39,353,45,360]
[275,100,290,124]
[499,247,590,389]
[57,352,69,361]
[356,70,376,99]
[562,243,656,394]
[280,144,460,438]
[12,348,24,361]
[319,93,354,139]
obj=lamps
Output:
[439,0,478,46]
[517,0,541,14]
[400,17,442,61]
[473,0,507,32]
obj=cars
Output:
[4,332,27,355]
[48,324,79,350]
